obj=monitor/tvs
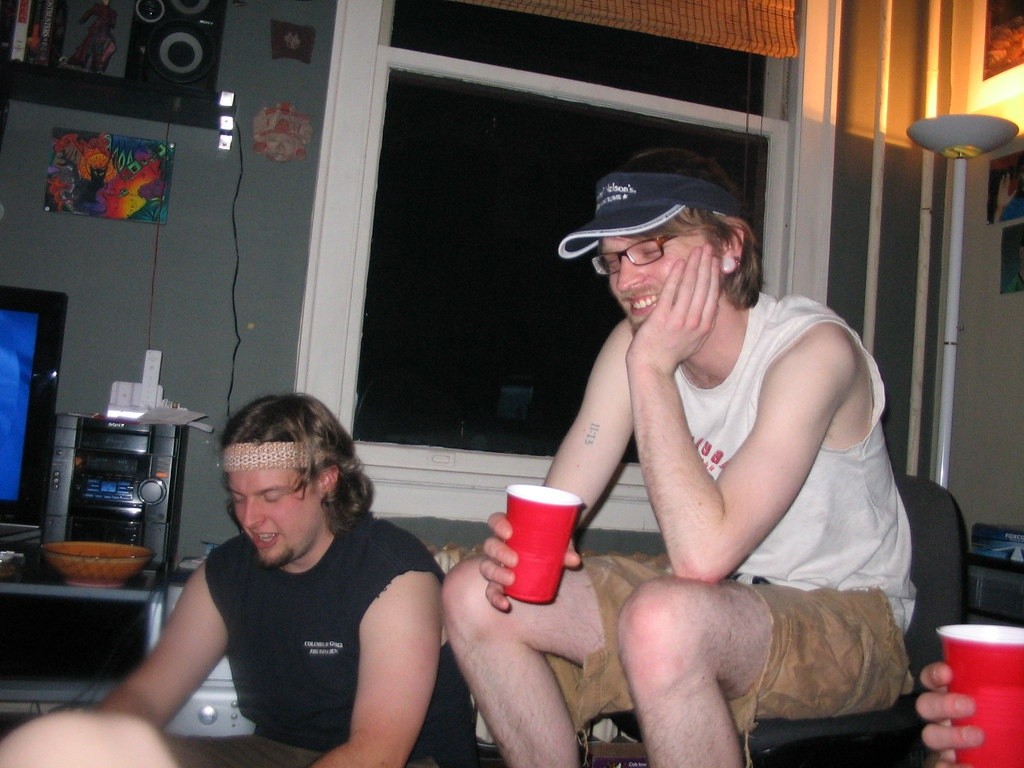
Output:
[0,284,68,544]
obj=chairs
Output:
[611,471,971,768]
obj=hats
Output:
[557,172,743,260]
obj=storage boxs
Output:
[0,539,170,706]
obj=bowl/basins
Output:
[40,540,156,587]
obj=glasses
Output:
[592,224,719,275]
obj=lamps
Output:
[906,114,1019,160]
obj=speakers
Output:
[124,0,228,101]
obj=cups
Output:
[935,625,1024,768]
[502,484,584,605]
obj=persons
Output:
[915,661,984,768]
[994,172,1024,223]
[444,146,916,768]
[0,709,177,768]
[100,392,477,768]
